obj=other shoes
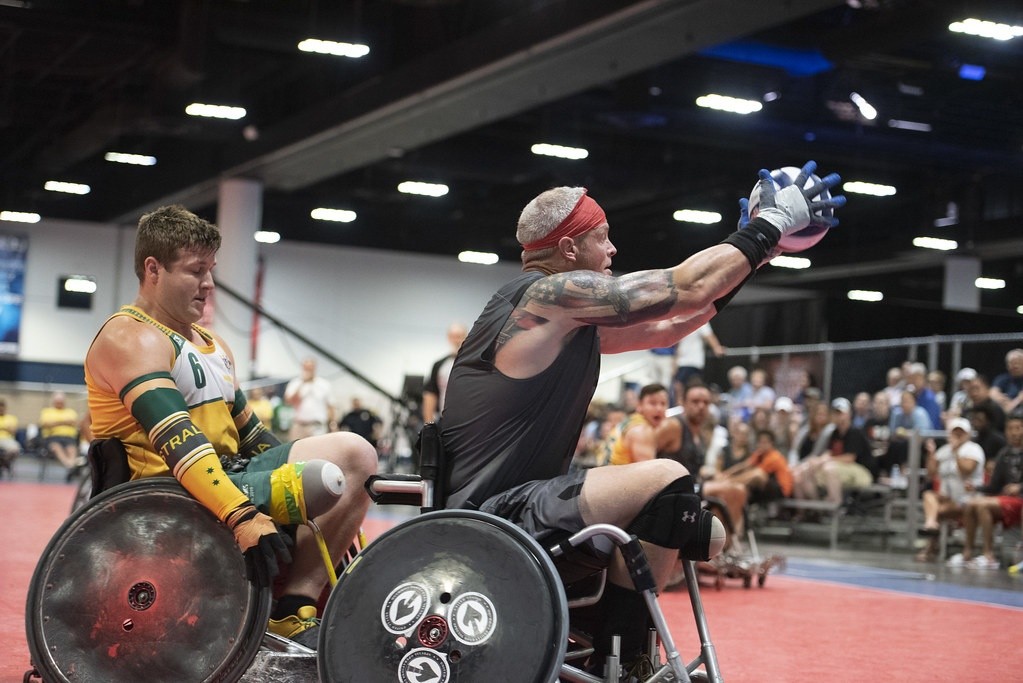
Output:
[918,528,940,536]
[67,467,80,482]
[944,553,967,568]
[968,556,1000,570]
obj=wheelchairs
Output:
[24,437,368,683]
[659,485,788,590]
[315,474,724,683]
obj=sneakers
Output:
[585,650,655,683]
[268,606,322,650]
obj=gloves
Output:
[227,505,295,588]
[757,161,847,237]
[738,198,782,269]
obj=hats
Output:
[957,367,977,381]
[774,397,792,413]
[908,363,925,375]
[947,418,970,433]
[832,397,850,413]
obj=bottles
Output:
[892,464,899,483]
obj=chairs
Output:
[30,431,63,482]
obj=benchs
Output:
[759,428,951,563]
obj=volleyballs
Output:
[747,166,834,253]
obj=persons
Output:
[576,348,1023,575]
[0,396,22,476]
[284,355,336,441]
[421,323,468,425]
[246,382,281,433]
[37,390,92,484]
[337,398,383,449]
[442,160,847,683]
[85,203,378,651]
